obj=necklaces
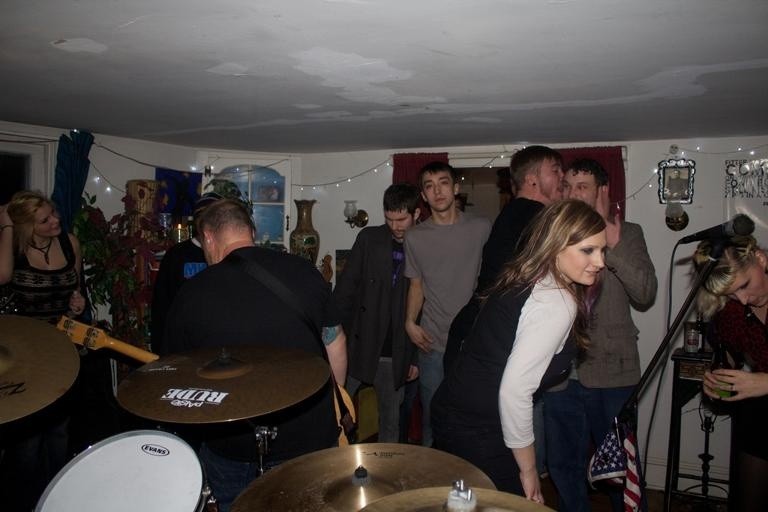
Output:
[27,238,52,265]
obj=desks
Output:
[664,346,737,511]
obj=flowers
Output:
[76,196,166,376]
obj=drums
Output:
[33,429,219,512]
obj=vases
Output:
[290,197,320,267]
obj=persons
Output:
[438,144,566,378]
[668,170,682,192]
[162,197,349,512]
[543,157,659,510]
[148,191,221,363]
[429,197,608,512]
[0,189,86,329]
[688,231,768,511]
[403,159,496,448]
[331,182,423,443]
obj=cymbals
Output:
[363,484,533,512]
[116,345,330,425]
[0,313,80,426]
[227,444,497,512]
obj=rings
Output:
[76,306,81,312]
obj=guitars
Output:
[53,315,357,450]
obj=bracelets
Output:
[0,224,15,230]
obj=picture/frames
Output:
[657,159,696,205]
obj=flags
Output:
[590,425,643,511]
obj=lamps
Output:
[663,199,689,232]
[344,199,369,229]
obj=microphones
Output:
[675,212,753,250]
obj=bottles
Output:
[173,223,187,245]
[683,320,698,353]
[187,215,194,239]
